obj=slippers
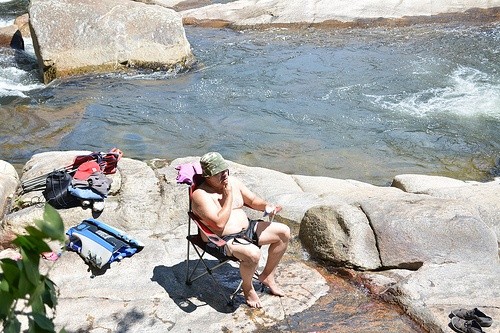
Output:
[452,308,492,327]
[451,316,486,333]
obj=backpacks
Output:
[42,169,79,209]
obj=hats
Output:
[199,152,229,177]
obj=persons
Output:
[191,151,291,308]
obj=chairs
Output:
[186,174,282,307]
